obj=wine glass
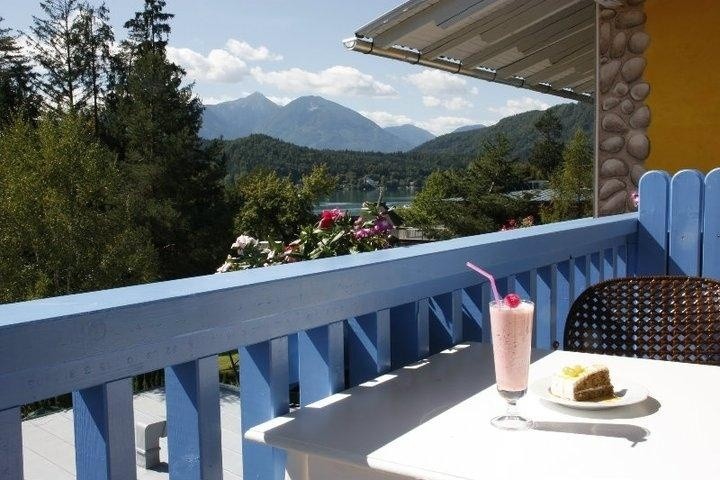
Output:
[488,297,536,429]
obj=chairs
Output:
[563,275,720,366]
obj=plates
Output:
[539,378,649,410]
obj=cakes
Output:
[551,363,615,401]
